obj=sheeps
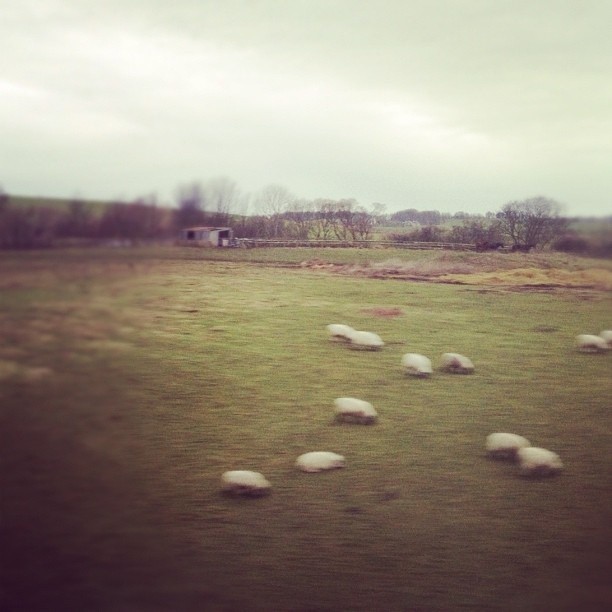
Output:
[330,397,378,424]
[441,352,474,373]
[295,451,346,474]
[220,470,273,498]
[574,334,610,353]
[600,330,612,345]
[347,330,386,348]
[326,321,354,342]
[400,352,433,376]
[486,432,530,459]
[516,446,564,475]
